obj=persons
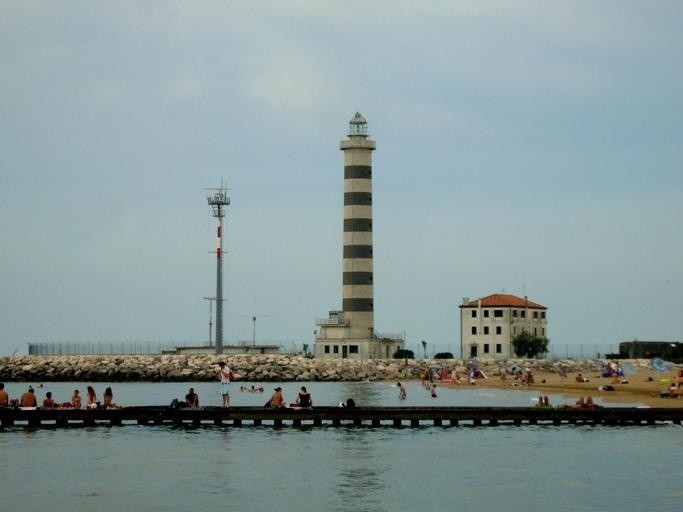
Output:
[294,386,313,406]
[239,384,263,393]
[536,393,603,408]
[504,368,536,385]
[269,386,286,406]
[420,365,482,385]
[184,387,198,408]
[216,361,231,408]
[396,382,406,400]
[430,385,437,397]
[0,383,118,408]
[576,374,583,382]
